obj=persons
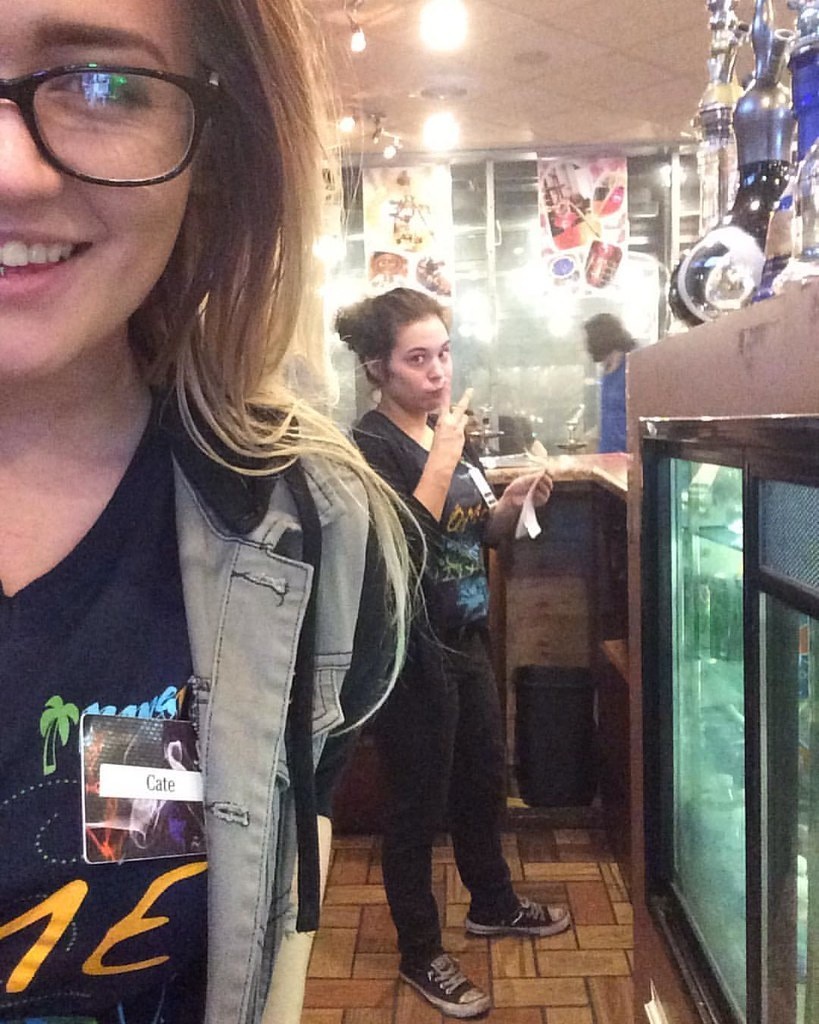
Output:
[584,313,640,455]
[0,0,429,1024]
[335,288,574,1013]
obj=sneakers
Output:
[466,894,572,938]
[397,946,493,1019]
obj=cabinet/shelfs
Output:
[642,438,760,1024]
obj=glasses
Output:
[0,62,235,187]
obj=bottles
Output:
[706,772,734,804]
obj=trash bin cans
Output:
[511,665,601,807]
